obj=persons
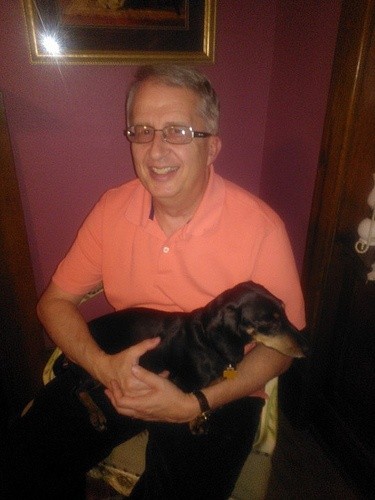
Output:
[0,65,306,500]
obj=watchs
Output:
[193,391,212,428]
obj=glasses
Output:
[126,125,212,144]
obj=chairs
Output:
[20,286,278,500]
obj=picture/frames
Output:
[22,0,217,62]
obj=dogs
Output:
[53,281,304,436]
